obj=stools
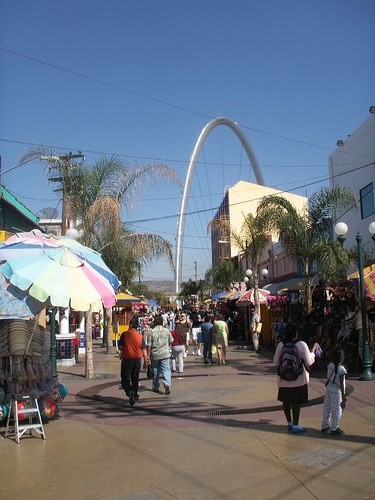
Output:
[4,393,46,444]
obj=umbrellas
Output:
[236,288,269,321]
[0,229,121,359]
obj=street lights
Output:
[46,227,83,380]
[333,221,375,382]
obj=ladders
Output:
[4,390,47,445]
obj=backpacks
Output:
[277,344,303,381]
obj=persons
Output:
[190,303,203,357]
[117,318,148,406]
[142,317,174,395]
[321,348,348,435]
[249,308,262,351]
[168,324,185,372]
[200,317,212,366]
[177,315,189,357]
[212,316,228,364]
[273,324,317,432]
[107,302,241,328]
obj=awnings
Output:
[210,290,246,301]
[265,276,321,294]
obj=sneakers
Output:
[321,427,346,435]
[288,421,306,433]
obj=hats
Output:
[100,303,257,321]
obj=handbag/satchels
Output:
[252,322,262,333]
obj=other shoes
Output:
[153,388,159,393]
[252,350,260,354]
[218,359,225,365]
[171,369,177,372]
[192,349,196,355]
[198,349,201,356]
[128,390,139,405]
[165,386,170,395]
[204,357,211,364]
[185,349,188,353]
[178,370,184,372]
